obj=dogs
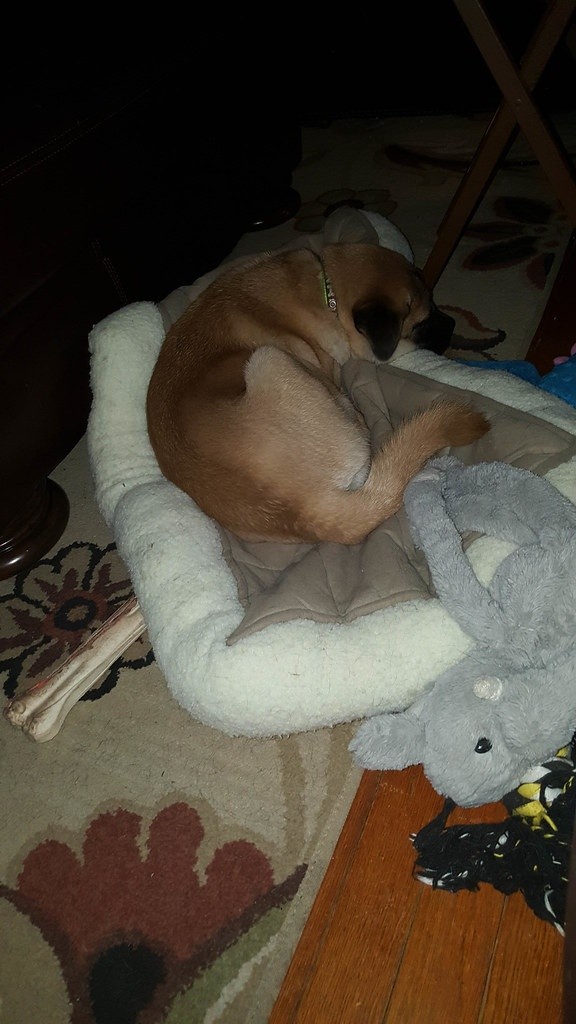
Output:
[146,239,495,545]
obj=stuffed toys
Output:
[345,457,576,808]
